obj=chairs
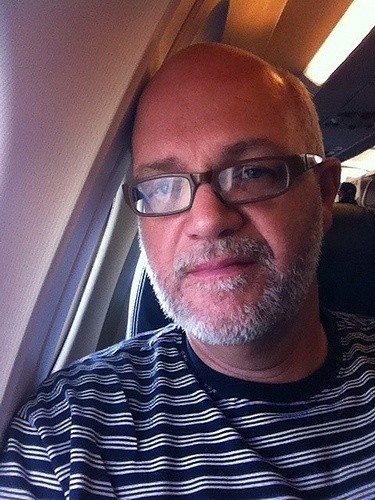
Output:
[130,201,375,340]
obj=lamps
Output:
[303,0,375,87]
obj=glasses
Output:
[121,153,325,215]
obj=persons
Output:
[1,36,374,499]
[335,182,358,205]
